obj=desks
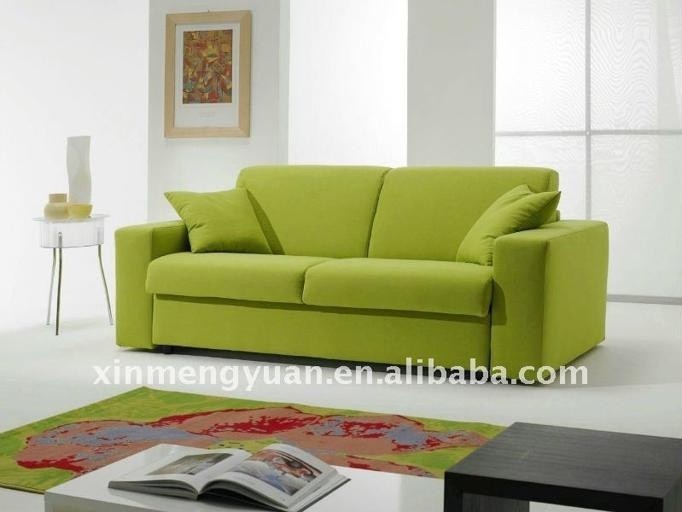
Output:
[40,437,451,510]
[29,210,116,337]
[442,422,682,511]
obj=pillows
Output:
[162,186,274,254]
[455,182,563,266]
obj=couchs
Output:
[112,161,611,385]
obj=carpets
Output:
[0,384,508,497]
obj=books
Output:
[107,444,351,512]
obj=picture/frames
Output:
[161,9,254,140]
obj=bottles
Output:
[44,193,68,219]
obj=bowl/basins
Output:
[69,203,94,218]
